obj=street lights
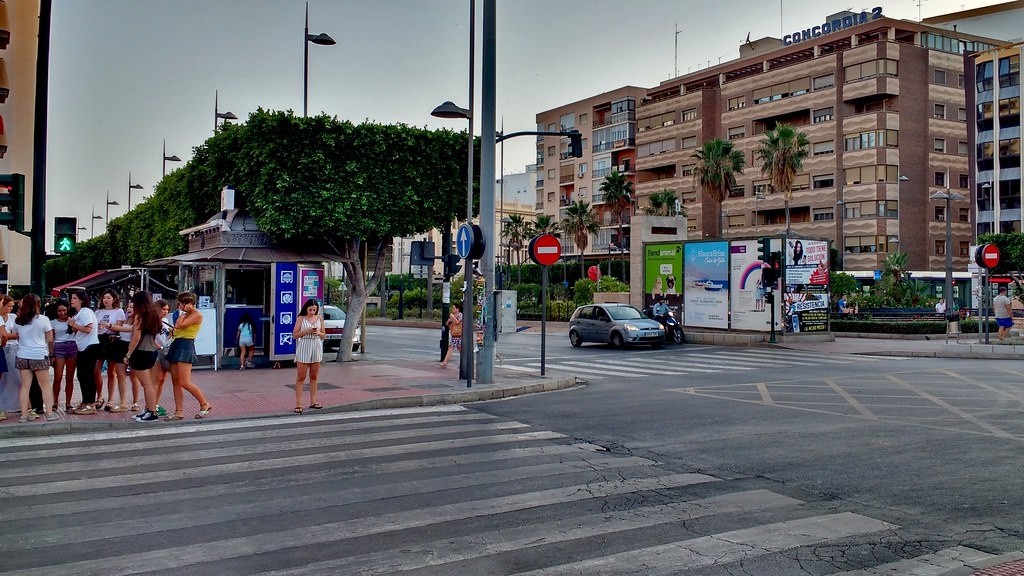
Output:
[163,155,181,178]
[91,216,103,239]
[214,108,239,134]
[431,100,475,382]
[106,201,119,224]
[304,33,337,118]
[930,189,967,315]
[77,227,88,243]
[129,184,144,212]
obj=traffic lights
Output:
[0,173,25,231]
[449,253,462,274]
[55,217,77,254]
[771,252,781,278]
[757,237,770,262]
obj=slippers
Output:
[309,403,322,409]
[164,415,184,421]
[294,407,303,413]
[195,406,212,419]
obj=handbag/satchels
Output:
[450,322,462,336]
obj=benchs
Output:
[580,312,594,318]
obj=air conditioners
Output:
[560,195,566,200]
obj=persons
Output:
[792,241,805,266]
[293,297,325,414]
[837,293,849,321]
[992,287,1014,341]
[755,279,767,312]
[439,303,463,369]
[652,296,674,328]
[0,289,212,422]
[495,325,505,366]
[235,312,256,370]
[935,298,946,317]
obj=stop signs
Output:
[534,235,562,266]
[982,243,999,268]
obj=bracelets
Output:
[159,346,164,350]
[74,324,77,328]
[72,323,75,326]
[179,310,188,317]
[109,324,113,328]
[125,354,131,360]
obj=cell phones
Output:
[312,328,317,330]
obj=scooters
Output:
[643,309,685,345]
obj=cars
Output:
[569,303,666,349]
[322,305,361,351]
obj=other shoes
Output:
[499,356,505,366]
[131,406,159,422]
[27,409,39,418]
[52,404,60,412]
[156,405,168,417]
[131,402,139,410]
[19,414,35,422]
[95,399,104,409]
[71,402,96,414]
[110,405,128,412]
[0,410,7,421]
[46,412,60,420]
[66,405,74,412]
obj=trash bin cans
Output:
[946,313,962,339]
